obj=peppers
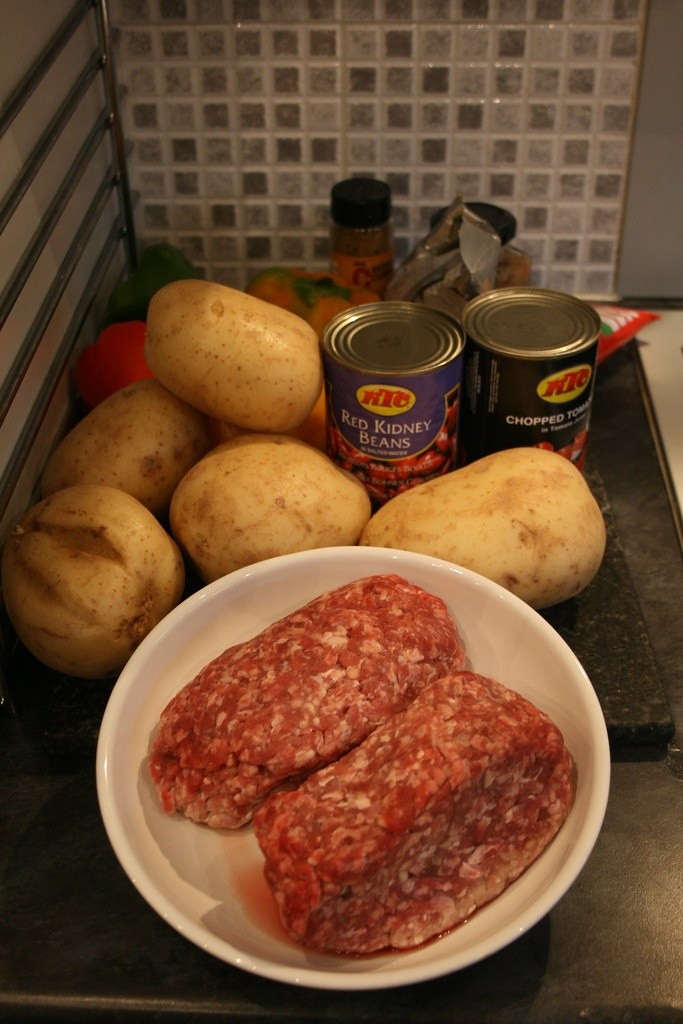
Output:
[74,242,205,411]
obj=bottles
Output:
[326,178,394,302]
[429,201,531,288]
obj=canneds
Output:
[329,178,394,292]
[321,302,466,506]
[462,287,601,472]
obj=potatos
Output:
[0,277,610,676]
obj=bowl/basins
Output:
[91,544,611,996]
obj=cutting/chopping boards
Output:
[3,430,674,749]
